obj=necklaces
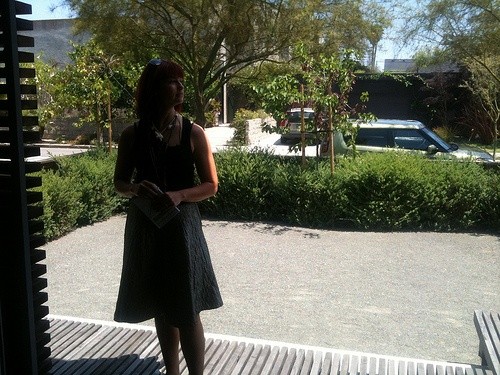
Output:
[147,113,180,192]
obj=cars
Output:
[280,108,319,145]
[320,120,494,170]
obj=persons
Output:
[112,58,224,375]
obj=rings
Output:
[140,190,145,194]
